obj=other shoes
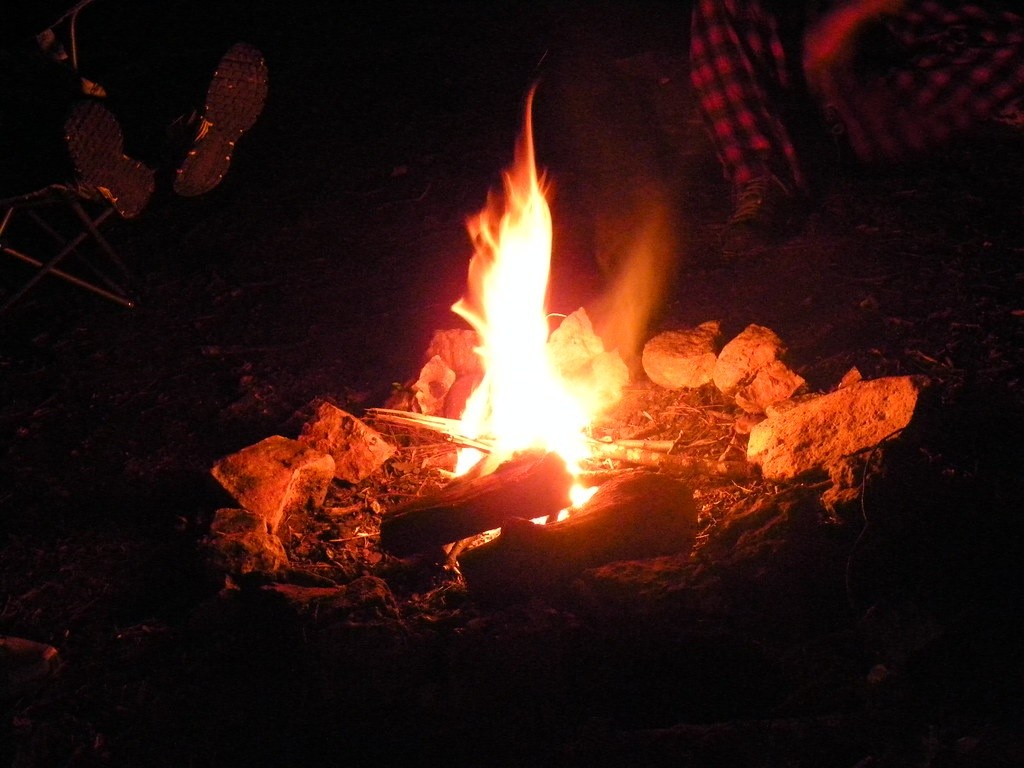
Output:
[172,43,269,196]
[715,176,799,257]
[64,101,157,219]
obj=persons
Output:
[1,636,58,691]
[4,1,270,223]
[673,2,1024,260]
